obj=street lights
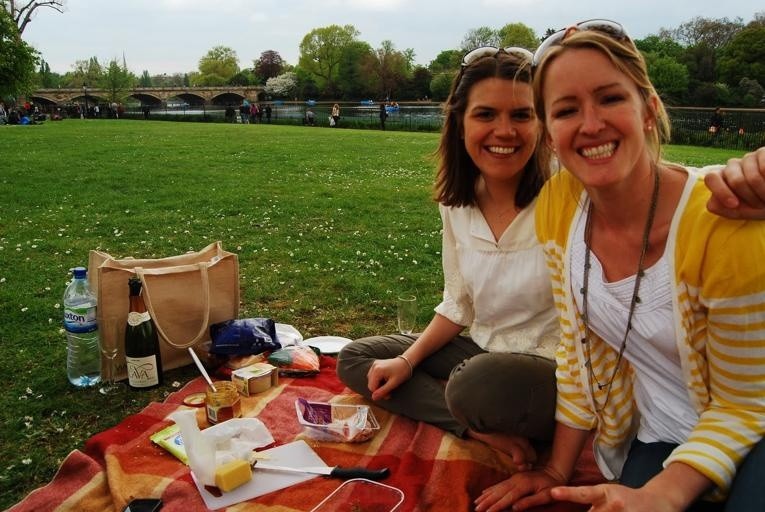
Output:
[81,81,88,117]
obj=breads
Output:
[215,461,252,493]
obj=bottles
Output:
[63,266,101,389]
[123,274,166,392]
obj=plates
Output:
[303,335,353,354]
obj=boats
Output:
[303,99,319,106]
[361,100,374,105]
[386,107,401,112]
[270,100,284,106]
[352,105,382,113]
[180,102,189,106]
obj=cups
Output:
[396,294,419,336]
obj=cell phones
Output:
[122,498,162,512]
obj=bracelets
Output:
[544,465,568,485]
[397,354,414,379]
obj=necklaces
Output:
[582,164,660,413]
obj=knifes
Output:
[252,463,392,482]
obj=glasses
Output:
[459,46,533,69]
[534,19,625,67]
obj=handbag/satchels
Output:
[89,241,240,383]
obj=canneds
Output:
[206,381,241,425]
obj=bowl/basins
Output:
[249,370,274,395]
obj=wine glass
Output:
[94,310,124,396]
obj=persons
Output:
[710,108,723,138]
[475,19,765,511]
[224,101,272,123]
[0,101,125,125]
[143,105,150,119]
[379,104,388,130]
[332,104,340,128]
[391,100,397,108]
[336,45,765,471]
[305,107,316,126]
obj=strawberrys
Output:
[335,498,361,511]
